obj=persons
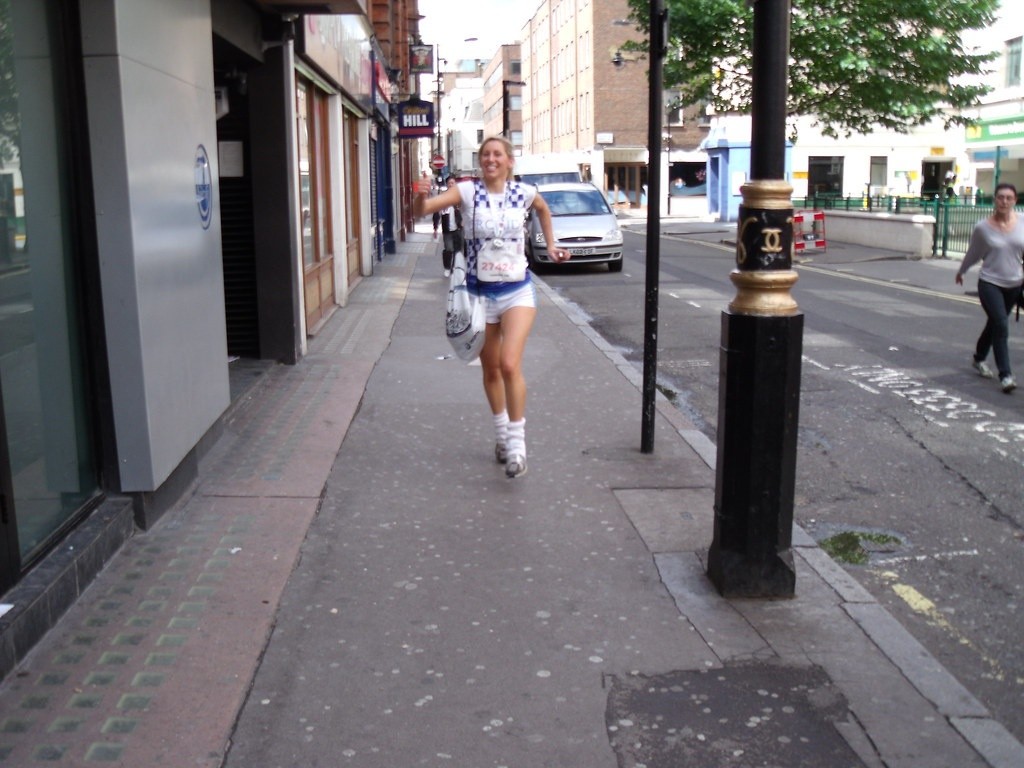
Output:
[421,139,571,478]
[955,184,1024,387]
[431,176,465,281]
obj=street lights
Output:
[614,47,673,215]
[436,33,481,153]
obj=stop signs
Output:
[431,155,445,169]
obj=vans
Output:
[509,154,587,206]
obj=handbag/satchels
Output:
[445,251,486,364]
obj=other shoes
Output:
[444,269,451,277]
[972,362,993,378]
[1002,377,1018,395]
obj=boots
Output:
[492,408,528,479]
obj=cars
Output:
[523,180,624,274]
